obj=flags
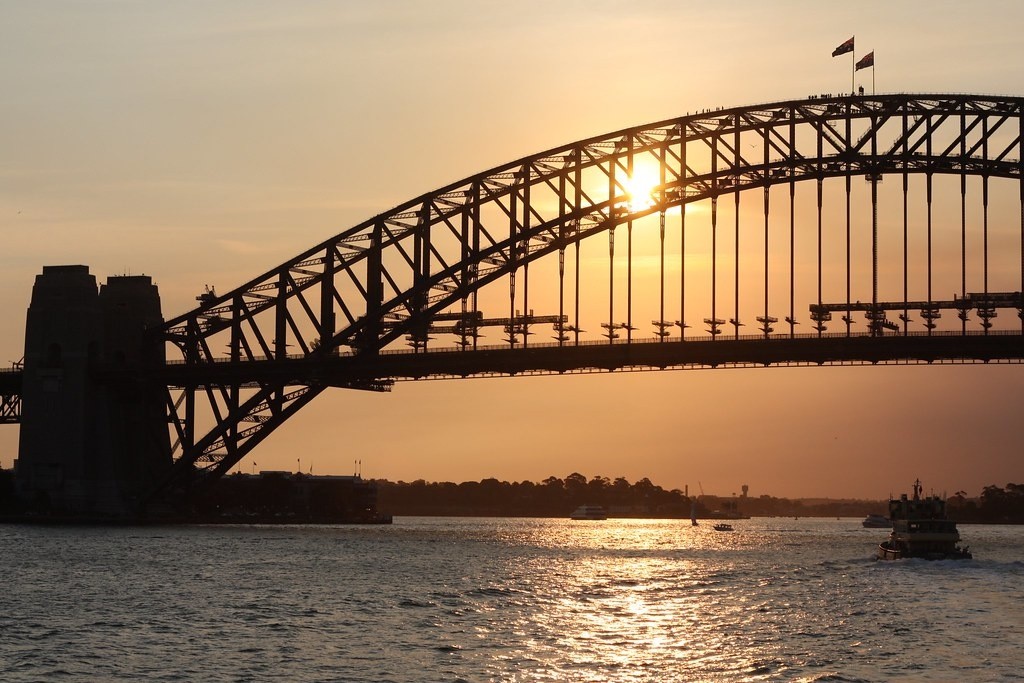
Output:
[856,52,873,70]
[832,36,854,57]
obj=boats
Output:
[713,523,733,532]
[878,478,973,561]
[862,514,892,529]
[569,505,608,521]
